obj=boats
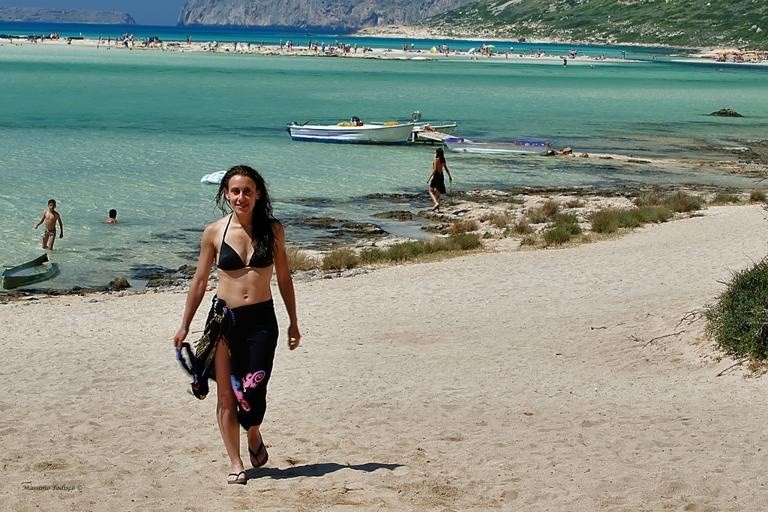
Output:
[197,169,227,185]
[0,251,60,291]
[286,122,553,155]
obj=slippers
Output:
[226,467,248,485]
[247,430,269,470]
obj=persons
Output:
[174,165,302,484]
[426,146,454,213]
[18,30,604,68]
[35,198,64,253]
[104,207,118,225]
